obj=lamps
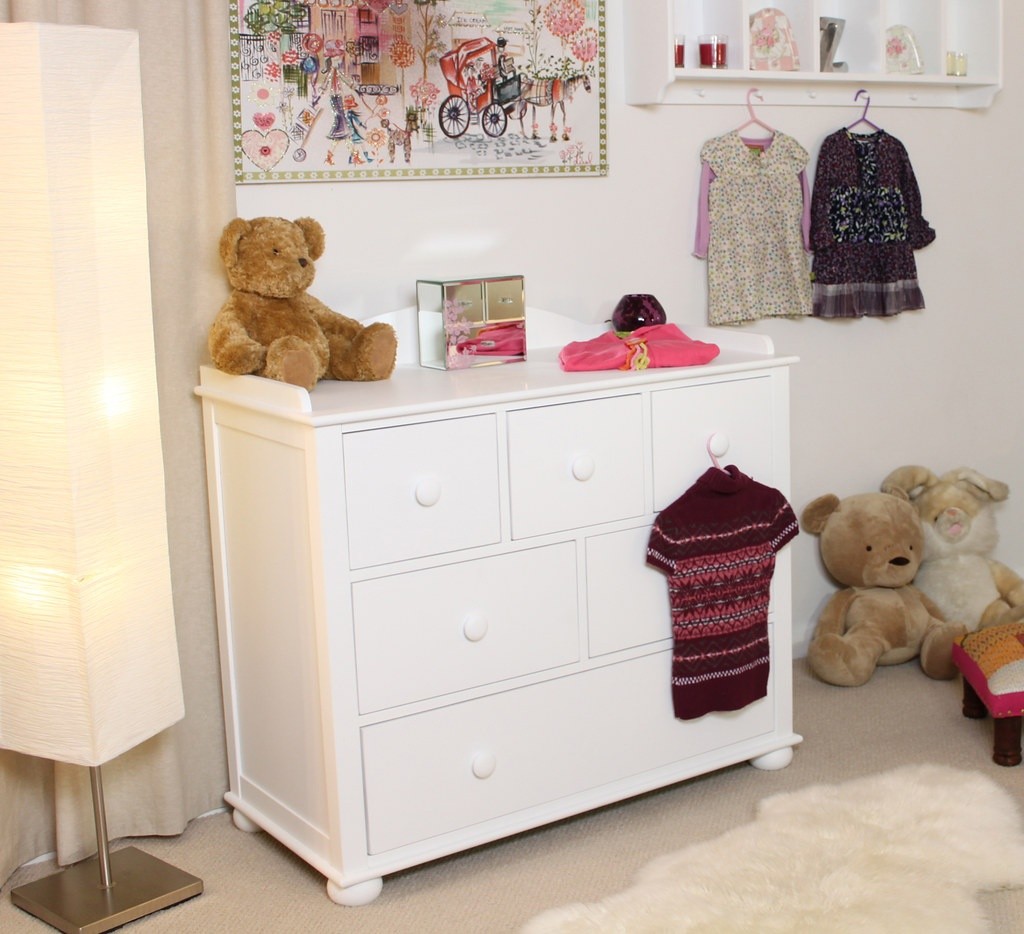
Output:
[0,17,209,934]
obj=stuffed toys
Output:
[802,483,966,686]
[209,215,399,390]
[879,466,1024,633]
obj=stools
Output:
[954,622,1024,767]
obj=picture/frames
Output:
[229,0,609,177]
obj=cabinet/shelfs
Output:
[624,0,1004,109]
[191,307,808,905]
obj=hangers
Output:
[738,88,773,132]
[847,89,881,131]
[707,432,731,476]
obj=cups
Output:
[945,51,967,77]
[673,34,685,67]
[699,34,729,69]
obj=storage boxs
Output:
[416,275,527,370]
